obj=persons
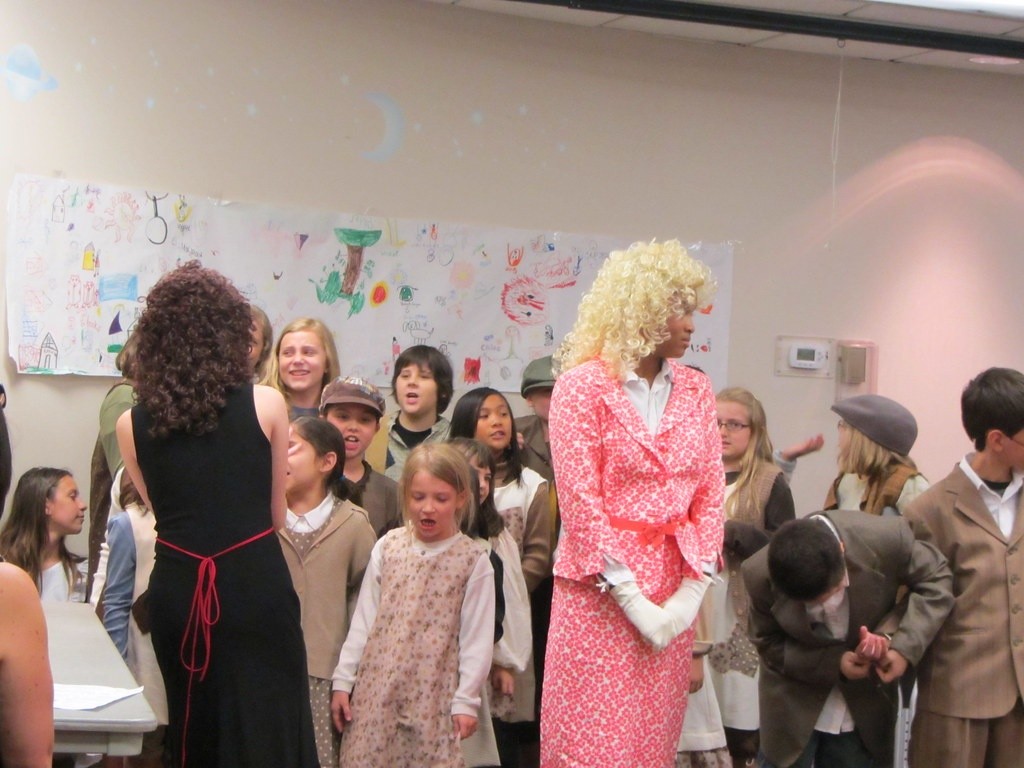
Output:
[741,509,955,768]
[685,365,824,768]
[319,375,401,537]
[450,356,562,768]
[331,442,495,768]
[116,259,322,768]
[539,239,734,768]
[102,468,170,768]
[825,395,929,768]
[277,417,378,768]
[852,367,1024,768]
[246,306,340,420]
[0,408,55,768]
[366,345,452,483]
[0,469,88,605]
[84,376,138,602]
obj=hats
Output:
[830,393,918,457]
[520,355,562,399]
[321,376,386,417]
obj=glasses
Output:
[802,557,849,611]
[717,418,752,433]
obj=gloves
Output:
[610,578,710,652]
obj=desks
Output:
[43,602,158,758]
